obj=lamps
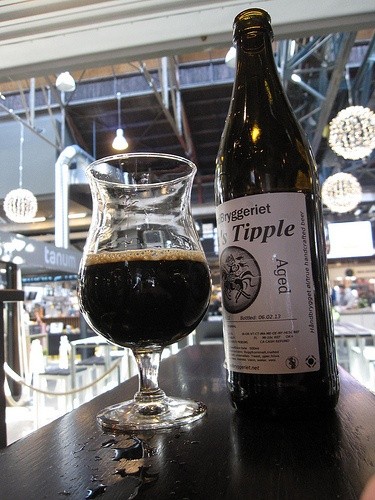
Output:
[3,120,38,223]
[329,106,375,160]
[320,172,362,214]
[224,45,238,67]
[112,92,128,151]
[55,72,75,93]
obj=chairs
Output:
[195,320,224,346]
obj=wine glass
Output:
[77,153,213,430]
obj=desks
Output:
[71,337,132,390]
[0,344,375,500]
[333,320,371,363]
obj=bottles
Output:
[214,9,340,416]
